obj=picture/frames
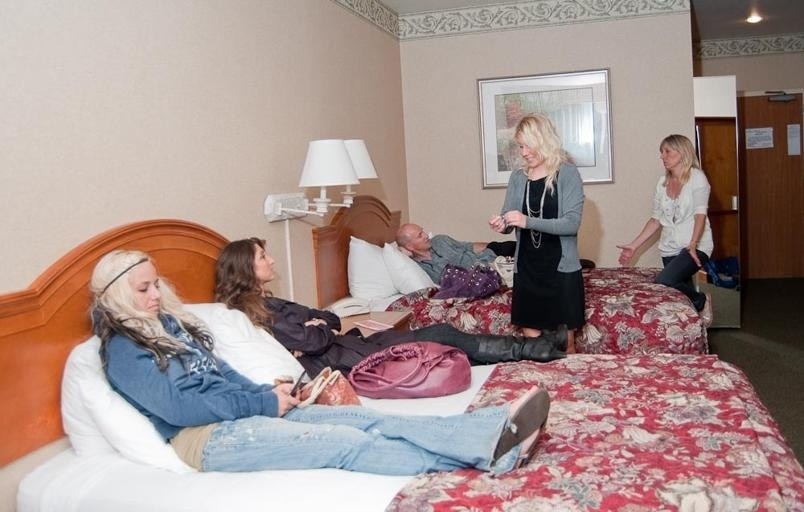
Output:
[476,66,615,190]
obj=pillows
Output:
[55,303,311,470]
[347,235,441,301]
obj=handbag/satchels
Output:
[296,366,362,410]
[348,341,472,399]
[432,263,501,297]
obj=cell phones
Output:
[290,370,307,395]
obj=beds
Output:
[312,195,710,354]
[0,219,804,512]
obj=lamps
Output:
[264,138,379,223]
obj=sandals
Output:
[698,293,714,329]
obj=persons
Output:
[89,249,551,473]
[616,135,713,328]
[216,238,567,380]
[396,224,594,286]
[489,113,584,355]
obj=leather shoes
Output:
[578,259,595,269]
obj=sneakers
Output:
[491,387,550,468]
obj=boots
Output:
[478,323,568,364]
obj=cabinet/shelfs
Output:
[693,76,741,328]
